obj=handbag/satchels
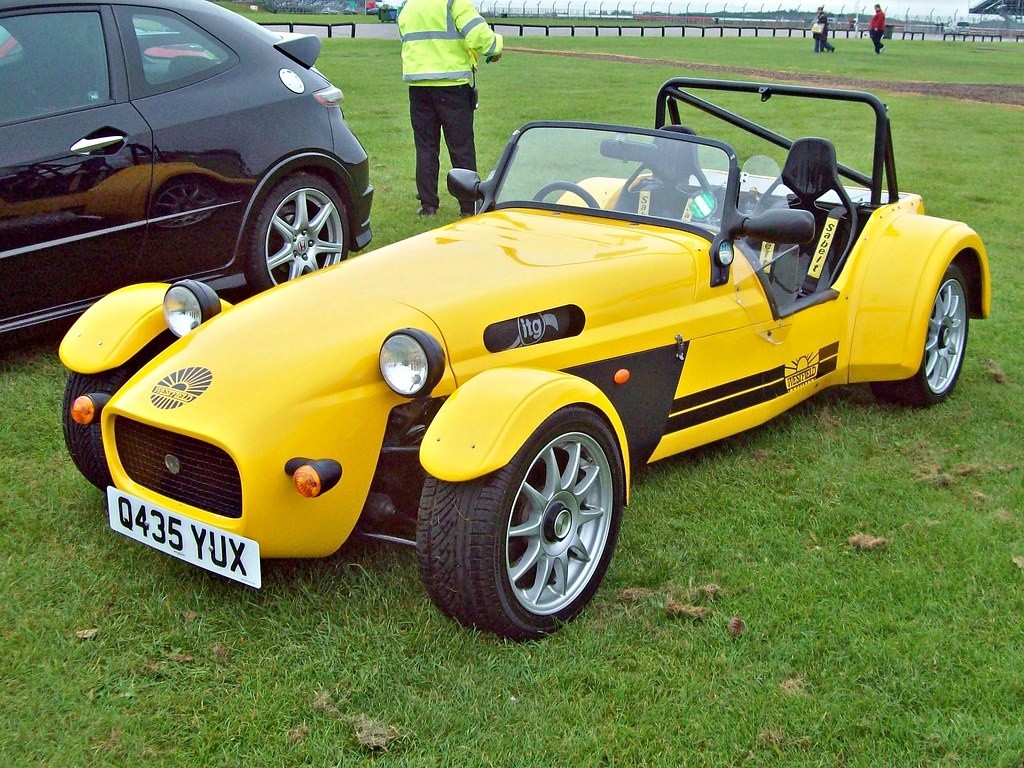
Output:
[811,14,825,34]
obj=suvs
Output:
[2,1,375,351]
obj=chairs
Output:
[613,125,721,225]
[741,136,858,300]
[164,55,217,82]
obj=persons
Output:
[935,22,945,34]
[812,7,835,53]
[871,4,885,56]
[395,0,504,218]
[366,1,375,11]
[849,17,855,31]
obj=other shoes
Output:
[416,206,435,215]
[460,205,474,216]
[879,46,885,53]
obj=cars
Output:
[57,77,993,646]
